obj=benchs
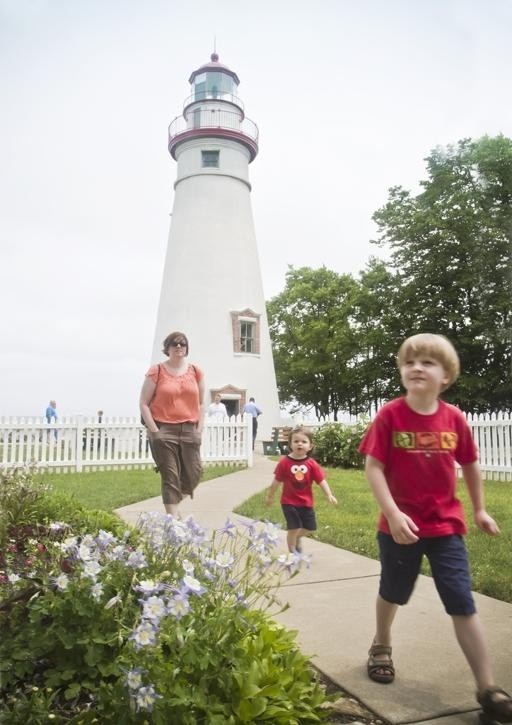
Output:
[262,426,293,456]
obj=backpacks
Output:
[142,365,199,427]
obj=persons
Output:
[96,410,104,425]
[266,427,337,564]
[359,333,510,725]
[39,399,58,444]
[139,331,204,518]
[241,397,262,451]
[207,393,228,422]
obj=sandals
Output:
[368,639,395,683]
[477,687,512,725]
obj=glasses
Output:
[171,341,187,346]
